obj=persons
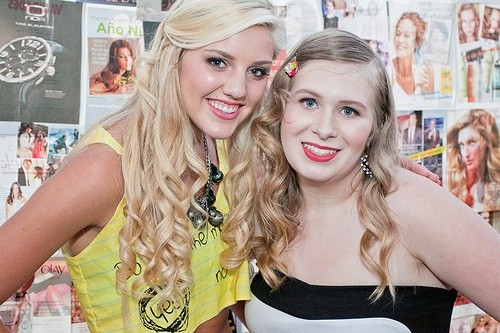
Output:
[6,0,500,333]
[244,28,500,333]
[0,0,284,333]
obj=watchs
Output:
[0,35,75,121]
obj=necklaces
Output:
[187,133,223,229]
